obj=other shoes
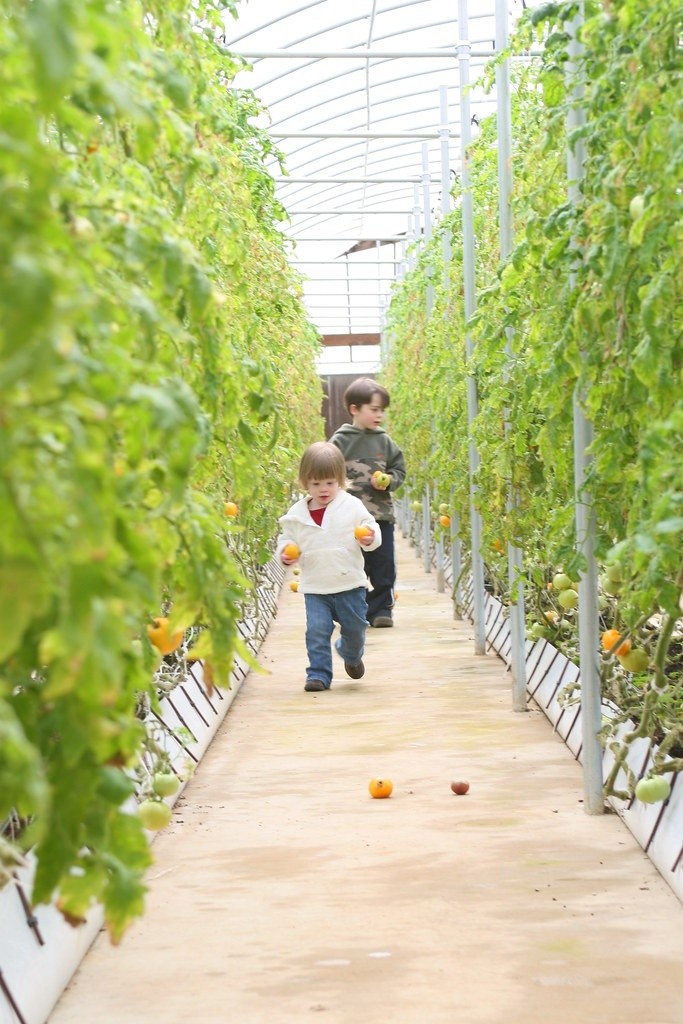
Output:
[305,679,326,690]
[371,610,394,628]
[336,643,365,680]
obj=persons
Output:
[277,441,382,692]
[328,377,407,628]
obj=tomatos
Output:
[368,777,393,798]
[140,469,391,657]
[135,768,179,831]
[409,500,649,674]
[635,775,669,803]
